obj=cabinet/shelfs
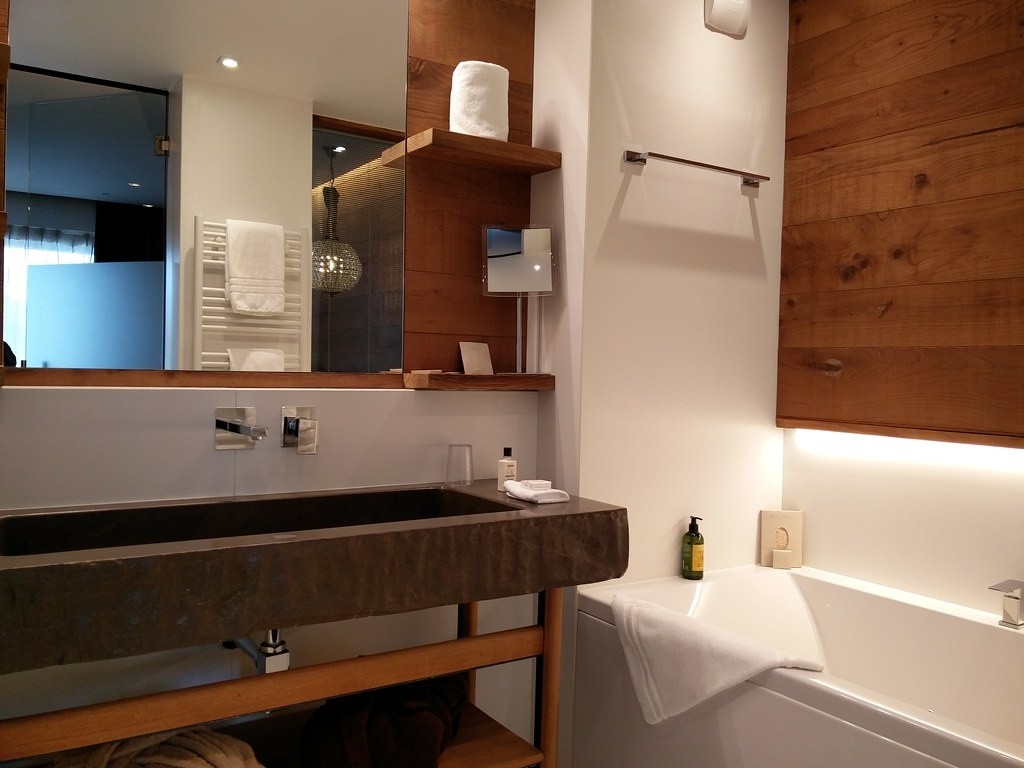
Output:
[404,127,563,393]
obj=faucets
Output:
[988,579,1024,630]
[228,420,267,440]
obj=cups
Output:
[448,444,474,486]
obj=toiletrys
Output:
[681,516,703,580]
[498,447,516,491]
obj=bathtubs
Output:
[571,564,1024,768]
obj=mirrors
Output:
[2,61,168,371]
[311,113,405,373]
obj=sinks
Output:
[1,479,631,679]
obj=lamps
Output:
[312,146,363,298]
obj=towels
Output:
[503,480,570,504]
[612,594,823,725]
[449,61,508,142]
[225,219,284,316]
[227,348,284,372]
